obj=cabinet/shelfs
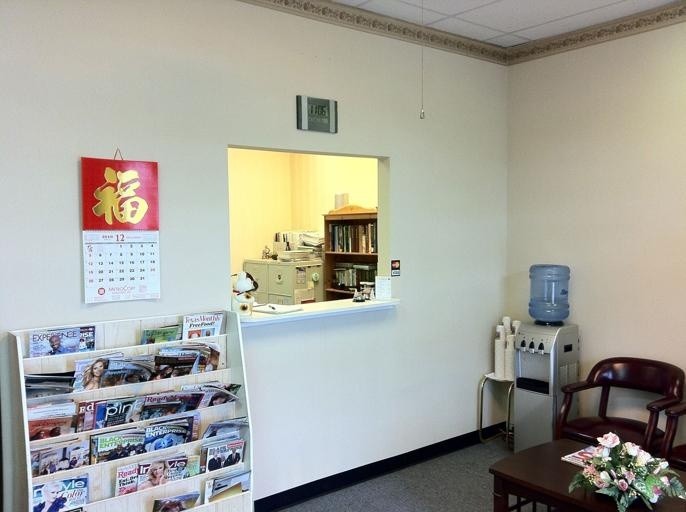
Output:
[4,309,255,512]
[242,258,322,306]
[323,211,378,302]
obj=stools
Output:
[477,373,515,450]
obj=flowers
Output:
[567,432,686,512]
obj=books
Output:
[561,444,597,469]
[275,229,324,264]
[328,217,379,292]
[252,303,303,315]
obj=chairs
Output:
[553,356,685,458]
[655,400,686,471]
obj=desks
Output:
[488,438,685,512]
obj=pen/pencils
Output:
[268,305,276,310]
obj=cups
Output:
[495,318,521,381]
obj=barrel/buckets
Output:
[527,264,571,323]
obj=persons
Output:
[24,314,253,512]
